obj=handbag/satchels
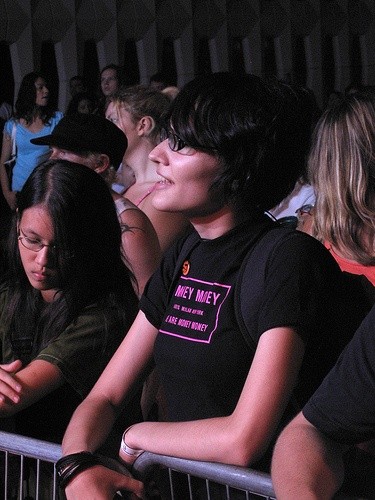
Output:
[3,156,15,192]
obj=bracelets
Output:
[56,452,99,489]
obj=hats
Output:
[30,113,128,166]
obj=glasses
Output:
[18,229,56,259]
[159,123,218,152]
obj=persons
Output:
[1,66,375,458]
[269,87,375,500]
[53,71,343,500]
